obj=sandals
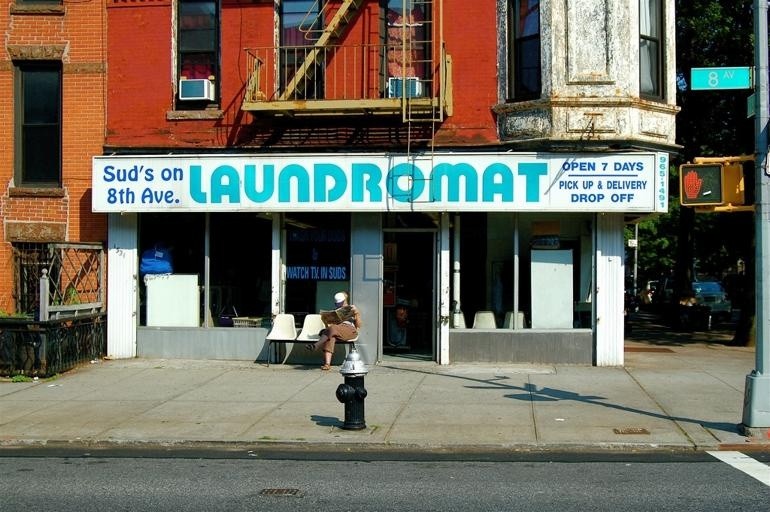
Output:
[305,345,319,352]
[321,364,330,370]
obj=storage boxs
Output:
[232,317,263,327]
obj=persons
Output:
[305,292,361,371]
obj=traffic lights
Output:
[678,162,747,209]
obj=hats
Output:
[334,292,345,304]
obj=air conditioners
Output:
[179,79,215,102]
[388,77,422,99]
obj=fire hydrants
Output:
[336,346,368,430]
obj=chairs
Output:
[265,314,359,367]
[450,311,528,329]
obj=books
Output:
[320,304,360,325]
